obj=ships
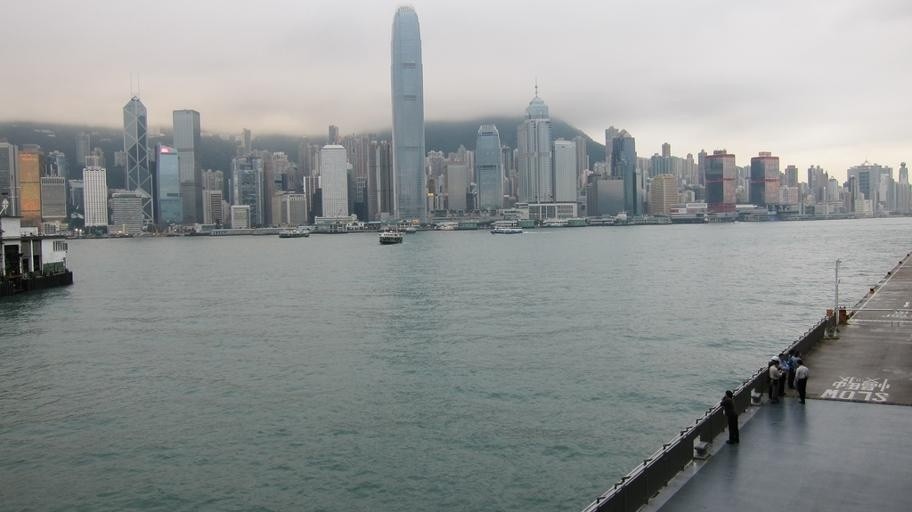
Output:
[578,247,911,512]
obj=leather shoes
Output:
[736,439,739,443]
[798,400,805,404]
[727,440,735,444]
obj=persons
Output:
[794,359,808,404]
[768,356,778,366]
[719,390,739,444]
[787,350,801,389]
[769,360,783,404]
[778,354,789,397]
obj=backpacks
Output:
[786,355,794,369]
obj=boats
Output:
[279,226,311,238]
[489,211,676,234]
[376,220,478,244]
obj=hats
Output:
[771,356,779,361]
